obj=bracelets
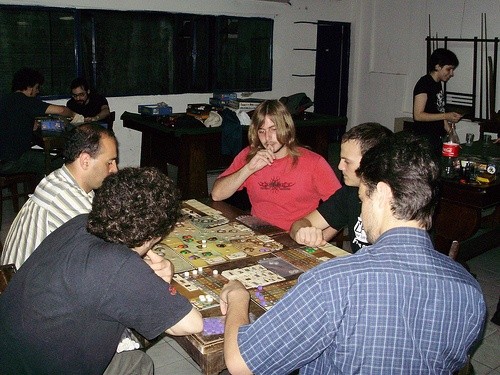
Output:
[96,115,100,121]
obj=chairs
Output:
[458,311,489,375]
[0,241,17,295]
[109,110,116,126]
[427,200,482,253]
[1,173,30,230]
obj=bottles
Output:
[442,123,460,178]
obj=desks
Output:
[120,110,349,199]
[431,141,500,265]
[134,195,354,375]
[40,117,112,175]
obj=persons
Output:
[0,68,76,173]
[0,168,203,375]
[219,130,487,375]
[67,78,110,122]
[413,49,462,150]
[211,100,343,243]
[290,121,394,253]
[0,124,117,269]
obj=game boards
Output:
[147,196,323,319]
